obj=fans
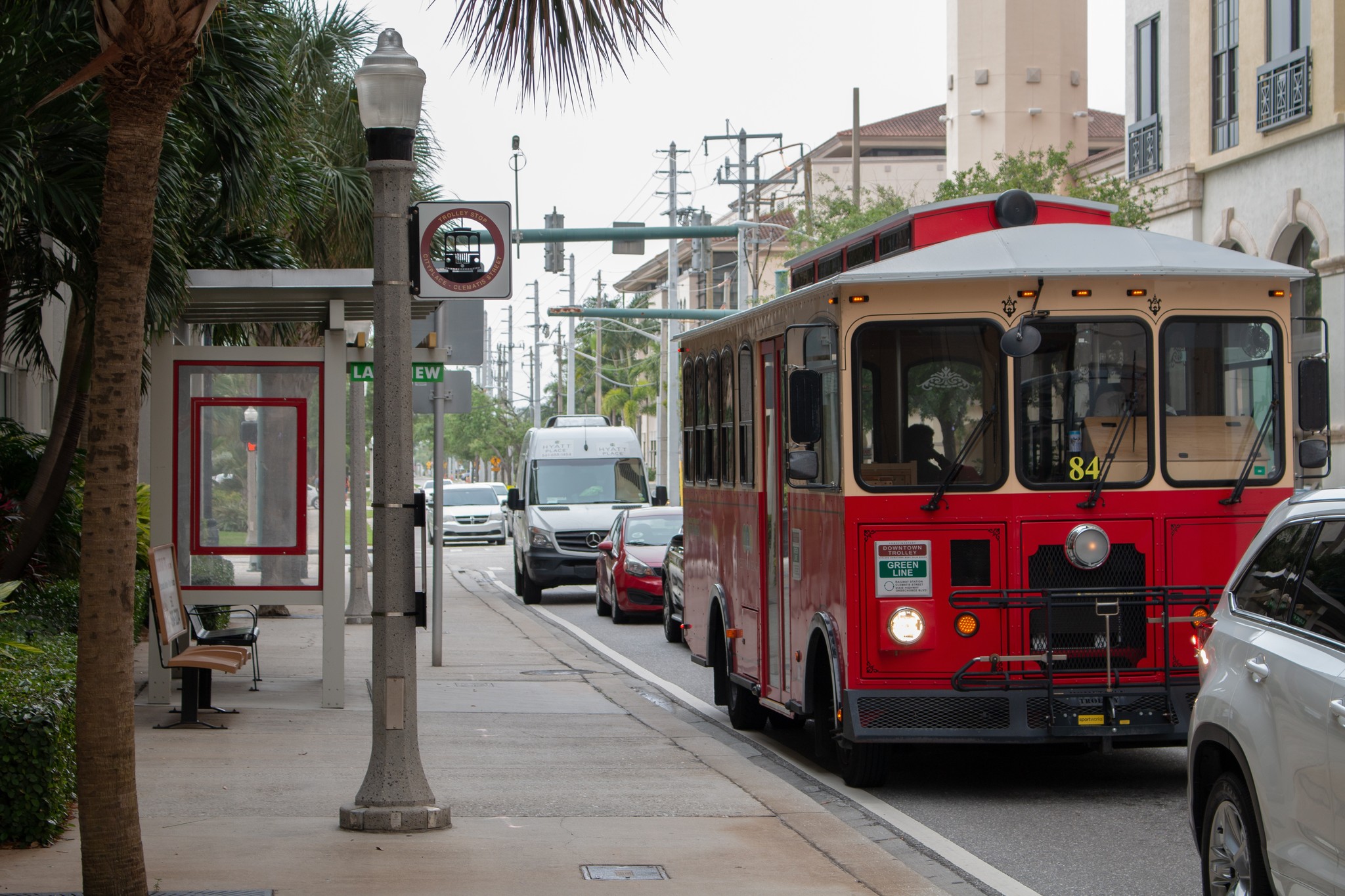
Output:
[1240,323,1270,358]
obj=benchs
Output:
[148,586,263,729]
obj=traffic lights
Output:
[246,440,258,452]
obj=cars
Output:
[596,507,683,625]
[413,478,511,547]
[290,483,319,509]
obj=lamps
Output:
[971,110,984,116]
[939,115,951,121]
[1029,108,1042,116]
[1073,111,1088,118]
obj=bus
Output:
[670,189,1332,788]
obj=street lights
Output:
[241,406,257,544]
[339,28,451,835]
[584,317,668,497]
[537,341,600,415]
[485,384,533,427]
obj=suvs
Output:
[1187,491,1344,896]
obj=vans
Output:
[506,414,667,604]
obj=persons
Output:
[903,424,954,486]
[312,476,319,495]
[1092,353,1178,416]
[345,476,350,506]
